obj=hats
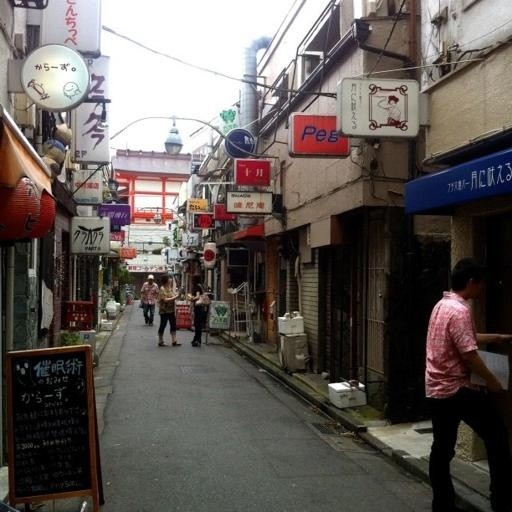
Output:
[147,274,154,279]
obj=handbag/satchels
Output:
[140,301,145,308]
[194,293,211,306]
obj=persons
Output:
[158,276,181,346]
[424,255,512,511]
[189,275,205,347]
[141,274,160,326]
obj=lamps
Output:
[164,119,183,156]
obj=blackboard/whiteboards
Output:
[1,345,98,504]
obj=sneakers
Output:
[145,317,153,325]
[159,341,167,346]
[192,339,201,346]
[172,340,181,345]
